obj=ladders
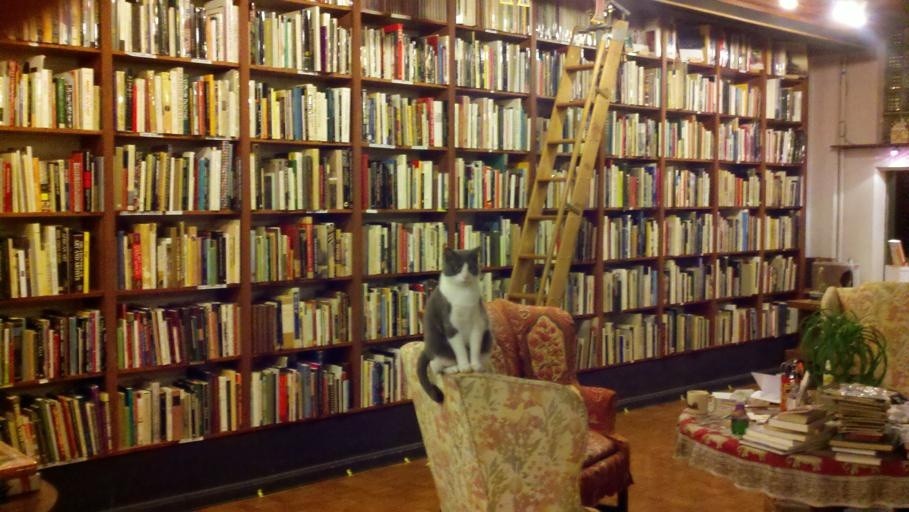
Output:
[501,22,630,307]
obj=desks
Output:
[678,402,909,512]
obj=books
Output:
[0,440,41,500]
[710,371,909,466]
[887,238,906,265]
[0,0,805,464]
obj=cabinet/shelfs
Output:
[0,2,810,512]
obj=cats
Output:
[415,244,494,404]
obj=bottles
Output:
[731,403,750,437]
[823,356,834,386]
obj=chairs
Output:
[819,281,909,402]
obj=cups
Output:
[686,390,716,415]
